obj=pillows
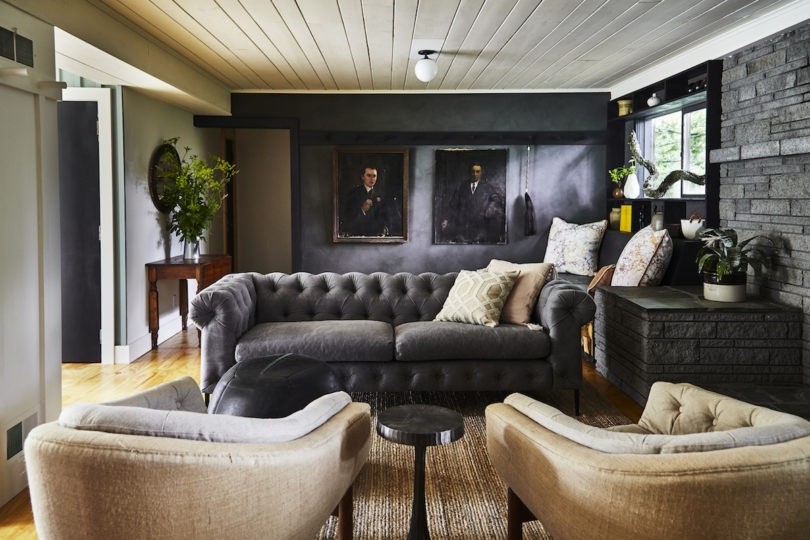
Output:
[434,269,522,327]
[544,216,674,292]
[487,259,558,331]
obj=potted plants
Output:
[154,137,240,259]
[608,157,638,199]
[696,228,779,303]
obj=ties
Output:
[367,189,374,198]
[472,182,477,192]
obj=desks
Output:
[375,405,465,540]
[593,284,803,409]
[145,255,232,350]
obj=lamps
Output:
[415,50,438,82]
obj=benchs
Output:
[530,221,706,363]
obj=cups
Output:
[620,205,632,231]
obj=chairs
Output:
[484,381,809,540]
[24,376,371,540]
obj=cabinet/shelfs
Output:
[607,59,723,243]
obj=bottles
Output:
[610,208,620,228]
[651,212,664,231]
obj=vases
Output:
[610,208,621,229]
[647,93,660,107]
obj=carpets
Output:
[316,377,636,540]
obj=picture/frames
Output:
[433,148,510,245]
[331,147,409,244]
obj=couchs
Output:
[189,272,598,414]
